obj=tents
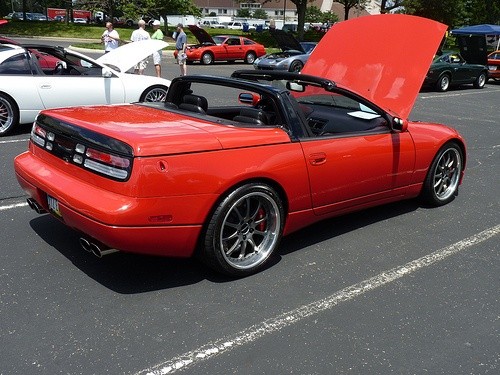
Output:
[450,23,500,36]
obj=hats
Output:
[152,20,160,25]
[175,23,183,27]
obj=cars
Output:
[10,11,53,22]
[282,22,332,36]
[0,30,174,136]
[122,16,155,27]
[172,23,268,65]
[53,14,87,24]
[13,13,471,272]
[488,49,500,80]
[200,19,270,29]
[254,25,321,73]
[417,51,488,91]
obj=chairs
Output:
[233,107,269,125]
[183,94,209,115]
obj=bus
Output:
[45,8,93,24]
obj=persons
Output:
[175,23,187,76]
[151,19,164,78]
[100,22,119,55]
[130,20,150,75]
